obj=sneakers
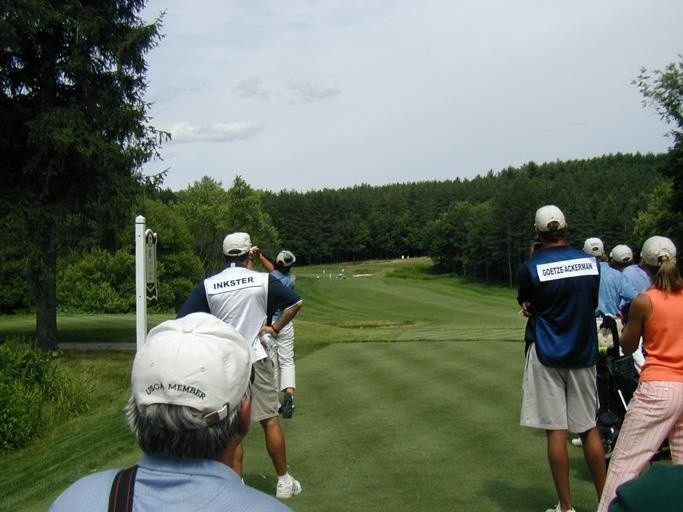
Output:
[545,500,576,512]
[278,391,296,418]
[276,472,302,499]
[571,437,583,446]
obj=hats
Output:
[640,235,677,267]
[534,205,566,232]
[582,237,633,262]
[276,250,297,267]
[222,232,251,257]
[130,312,254,429]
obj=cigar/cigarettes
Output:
[517,303,531,316]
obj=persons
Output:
[526,237,682,470]
[247,244,297,419]
[516,204,608,512]
[173,232,303,499]
[46,310,295,510]
[596,234,682,511]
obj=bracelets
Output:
[272,325,278,334]
